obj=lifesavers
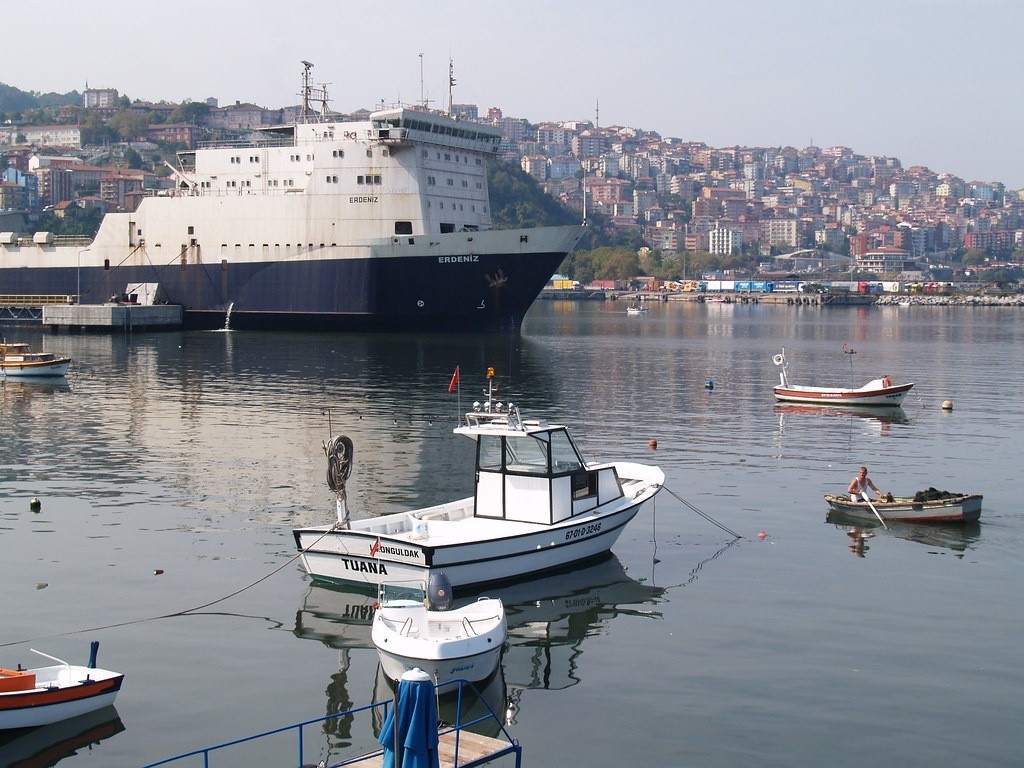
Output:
[350,132,356,139]
[773,353,785,366]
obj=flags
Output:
[370,538,378,557]
[449,367,458,392]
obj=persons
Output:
[847,467,884,503]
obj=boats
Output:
[293,553,668,690]
[1,59,588,331]
[771,347,915,407]
[898,301,915,306]
[372,662,508,746]
[0,374,72,394]
[0,640,125,730]
[627,307,649,313]
[292,368,665,602]
[773,401,909,433]
[825,508,980,551]
[0,705,126,768]
[373,574,509,698]
[0,339,71,378]
[824,486,983,525]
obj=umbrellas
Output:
[377,666,440,768]
